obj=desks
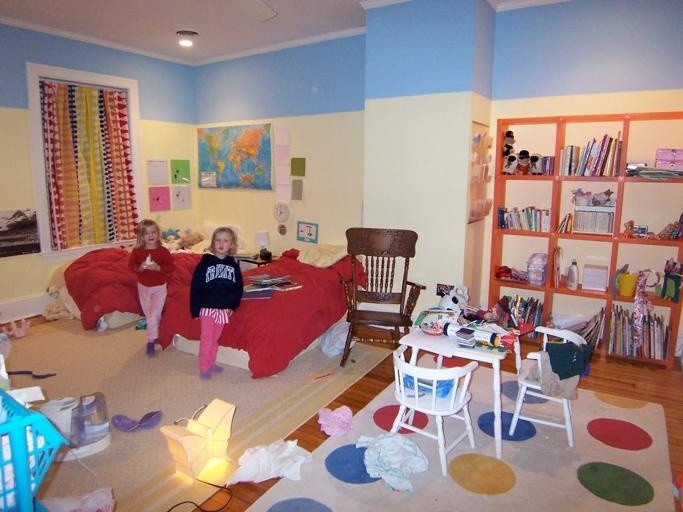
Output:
[399,320,523,460]
[237,257,280,270]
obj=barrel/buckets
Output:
[619,271,639,297]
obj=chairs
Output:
[388,326,588,477]
[338,228,426,367]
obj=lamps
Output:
[160,399,236,478]
[254,231,270,260]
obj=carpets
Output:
[3,317,393,511]
[245,354,677,512]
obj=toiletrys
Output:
[567,259,579,290]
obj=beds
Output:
[57,234,248,328]
[173,247,361,377]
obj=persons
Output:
[189,227,244,382]
[128,219,176,358]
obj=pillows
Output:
[191,239,244,255]
[298,243,347,269]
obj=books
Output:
[558,132,623,178]
[240,275,304,301]
[498,206,550,232]
[555,214,571,235]
[609,303,671,362]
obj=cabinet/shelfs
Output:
[487,112,683,369]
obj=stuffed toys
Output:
[503,131,538,175]
[465,304,497,321]
